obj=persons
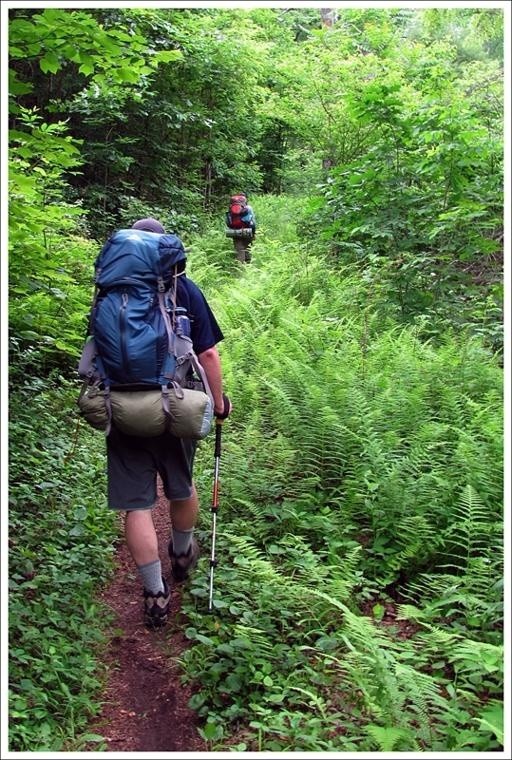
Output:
[222,192,259,267]
[65,215,233,629]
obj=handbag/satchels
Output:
[78,390,213,440]
[225,229,254,240]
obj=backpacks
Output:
[225,195,255,229]
[78,229,215,389]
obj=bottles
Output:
[167,305,192,339]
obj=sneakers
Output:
[143,576,171,627]
[168,538,199,580]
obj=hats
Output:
[132,219,166,235]
